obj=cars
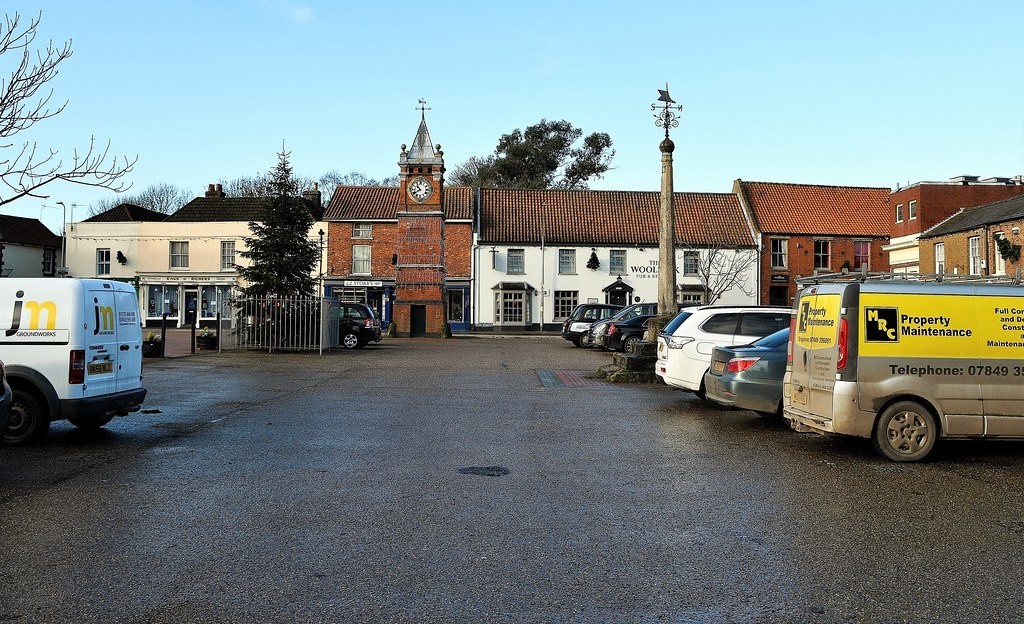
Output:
[562,303,703,352]
[704,324,794,428]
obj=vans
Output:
[781,265,1023,463]
[0,278,147,443]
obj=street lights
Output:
[318,229,326,298]
[55,201,67,280]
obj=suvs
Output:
[655,303,792,410]
[338,302,383,350]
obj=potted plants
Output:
[586,250,600,269]
[388,323,395,338]
[116,250,127,265]
[196,325,218,350]
[840,260,851,271]
[440,322,450,339]
[993,233,1023,264]
[141,331,165,357]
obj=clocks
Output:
[406,176,435,204]
